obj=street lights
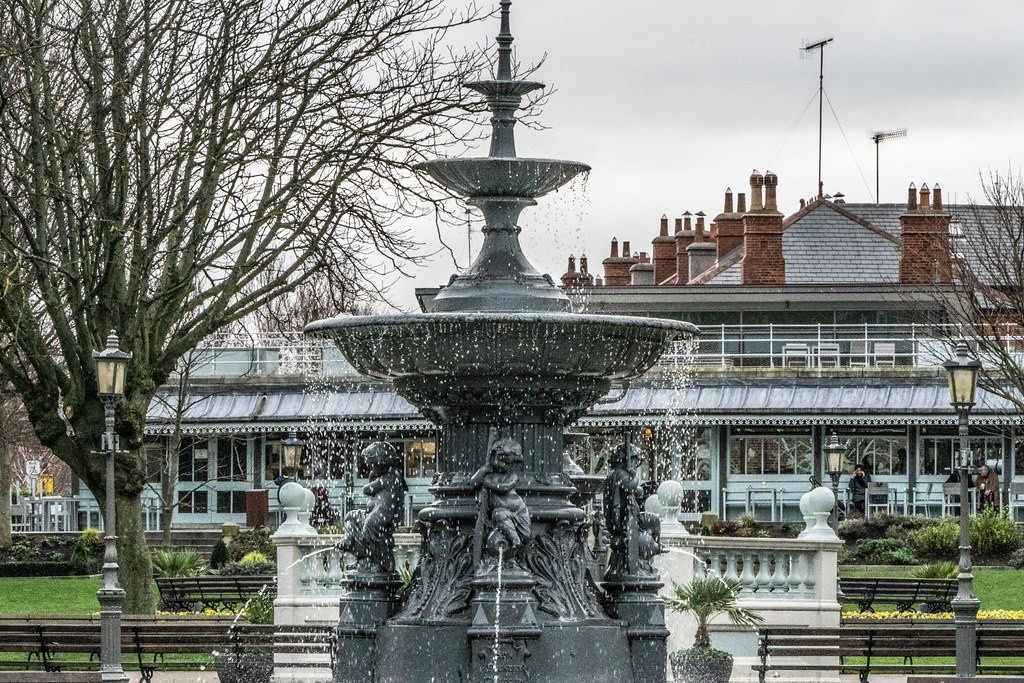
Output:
[941,337,982,676]
[91,328,132,683]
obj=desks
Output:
[782,347,811,369]
[864,488,898,516]
[812,346,840,369]
[943,486,980,517]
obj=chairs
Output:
[782,342,897,371]
[843,487,855,517]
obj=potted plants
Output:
[660,573,764,683]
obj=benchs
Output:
[154,576,278,614]
[0,614,339,683]
[751,620,1024,683]
[835,575,959,613]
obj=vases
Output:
[212,653,274,683]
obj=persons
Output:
[974,460,1000,512]
[945,470,975,516]
[469,437,533,551]
[332,432,409,571]
[848,463,871,513]
[602,443,671,555]
[893,447,908,475]
